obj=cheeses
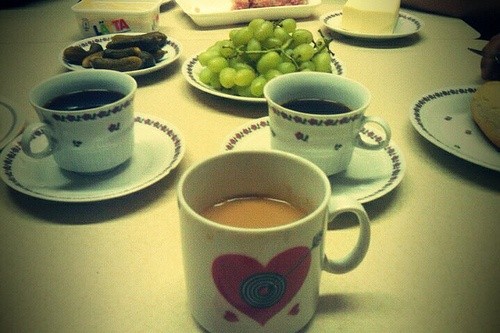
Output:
[341,0,401,34]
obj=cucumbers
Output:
[63,32,167,71]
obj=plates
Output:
[181,45,345,102]
[0,95,26,153]
[0,113,184,203]
[179,0,321,27]
[58,33,184,76]
[320,10,422,40]
[409,84,500,172]
[219,116,405,202]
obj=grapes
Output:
[198,19,333,97]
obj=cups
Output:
[263,71,391,177]
[21,69,138,175]
[176,149,371,332]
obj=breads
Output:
[472,80,500,152]
